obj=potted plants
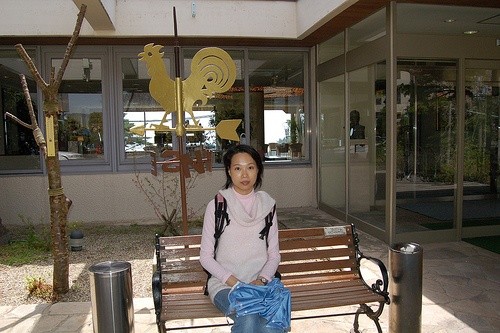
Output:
[288,113,303,152]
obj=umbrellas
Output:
[225,278,291,332]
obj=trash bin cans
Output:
[386,241,422,333]
[88,260,136,333]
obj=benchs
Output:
[152,223,390,333]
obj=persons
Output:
[200,144,292,333]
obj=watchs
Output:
[257,277,268,285]
